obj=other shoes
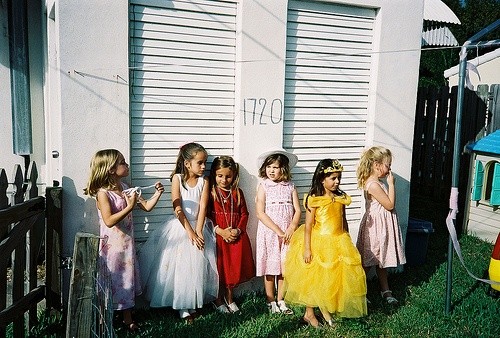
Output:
[320,314,337,327]
[303,317,324,328]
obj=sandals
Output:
[381,290,399,306]
[209,301,230,314]
[266,301,281,315]
[277,301,294,315]
[224,295,242,315]
[178,309,193,325]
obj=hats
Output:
[256,148,298,173]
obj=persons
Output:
[356,146,407,306]
[206,155,256,315]
[83,149,164,334]
[255,150,302,315]
[282,159,368,329]
[137,142,220,324]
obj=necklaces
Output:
[218,187,233,230]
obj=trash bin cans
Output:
[405,217,435,269]
